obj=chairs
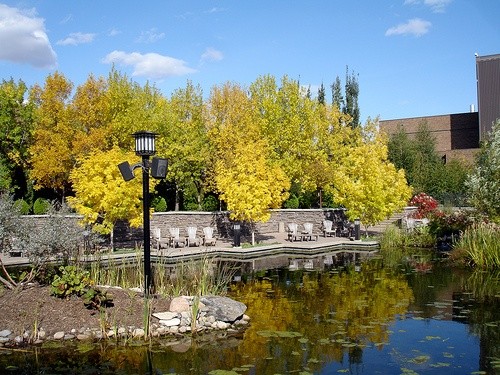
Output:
[199,227,217,246]
[185,227,203,246]
[150,229,171,249]
[168,227,187,247]
[323,220,337,238]
[287,222,318,243]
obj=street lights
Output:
[117,130,169,292]
